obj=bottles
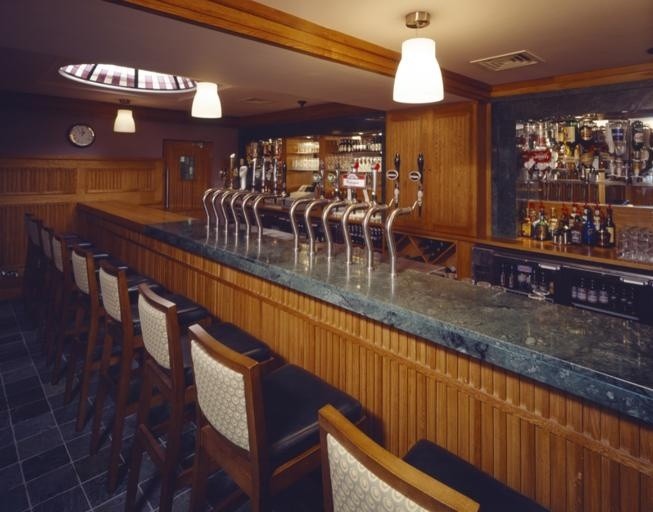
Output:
[338,136,382,152]
[325,156,381,172]
[418,237,445,260]
[570,275,608,306]
[497,265,515,289]
[520,201,617,245]
[556,117,643,181]
[340,223,385,246]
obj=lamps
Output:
[112,97,136,133]
[392,10,445,107]
[189,79,223,119]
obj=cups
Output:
[617,226,652,263]
[292,142,319,169]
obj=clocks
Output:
[67,122,96,148]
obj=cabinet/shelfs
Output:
[240,128,381,222]
[468,233,652,324]
[386,101,486,236]
